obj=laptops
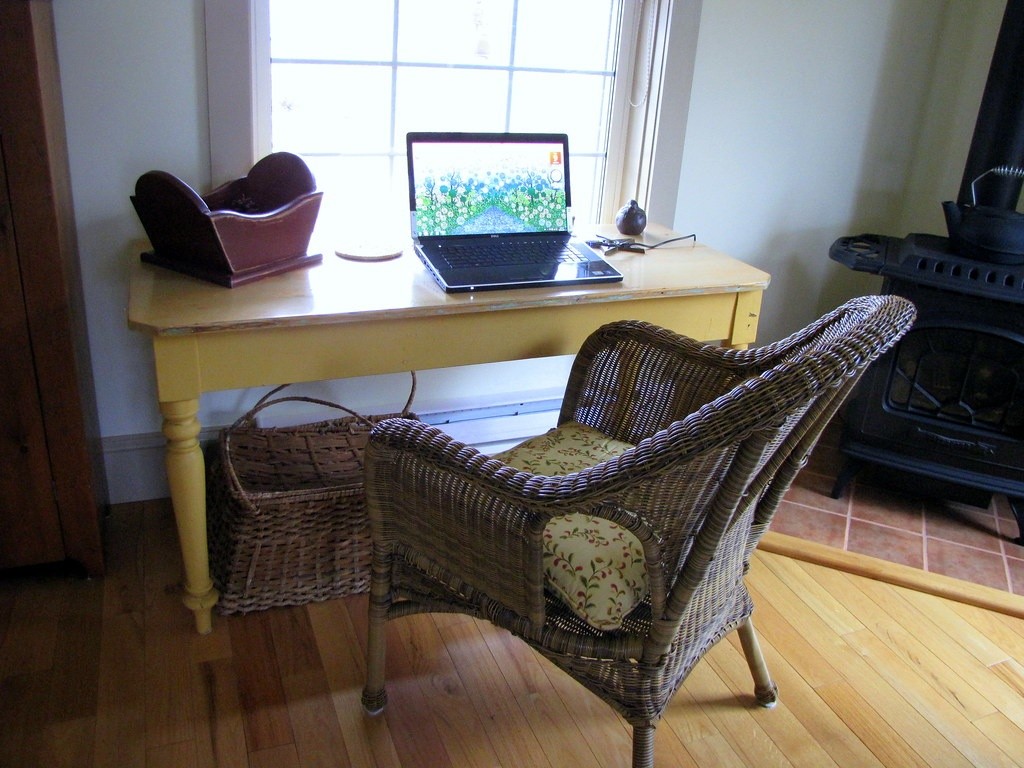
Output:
[406,132,624,293]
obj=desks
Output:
[128,223,771,635]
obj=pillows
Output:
[442,418,711,631]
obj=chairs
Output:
[360,295,916,768]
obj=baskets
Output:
[205,369,422,617]
